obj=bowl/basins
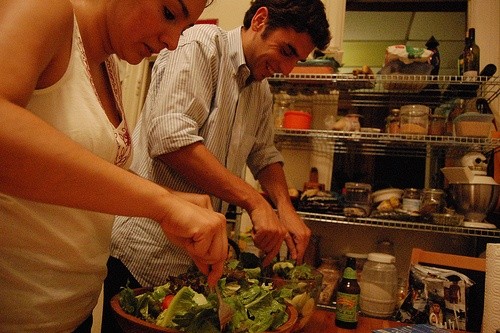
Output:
[431,213,464,226]
[448,182,499,221]
[223,266,325,296]
[372,189,404,204]
[454,111,493,138]
[109,286,299,333]
[282,110,312,130]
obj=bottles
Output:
[387,108,401,137]
[375,239,396,256]
[335,257,361,329]
[457,29,469,80]
[302,235,322,268]
[358,253,398,319]
[447,98,468,135]
[400,187,421,217]
[419,187,446,223]
[316,256,342,305]
[463,28,480,81]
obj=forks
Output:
[215,282,235,330]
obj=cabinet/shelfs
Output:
[266,73,500,239]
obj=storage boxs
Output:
[290,60,338,73]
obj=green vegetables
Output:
[118,253,322,333]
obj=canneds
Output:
[429,114,448,136]
[386,109,402,134]
[402,189,447,218]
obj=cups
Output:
[400,105,429,135]
[344,182,371,216]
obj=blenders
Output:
[448,155,497,229]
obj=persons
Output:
[1,1,228,333]
[101,0,332,333]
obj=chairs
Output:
[410,248,486,333]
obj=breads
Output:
[332,115,361,132]
[384,47,431,64]
